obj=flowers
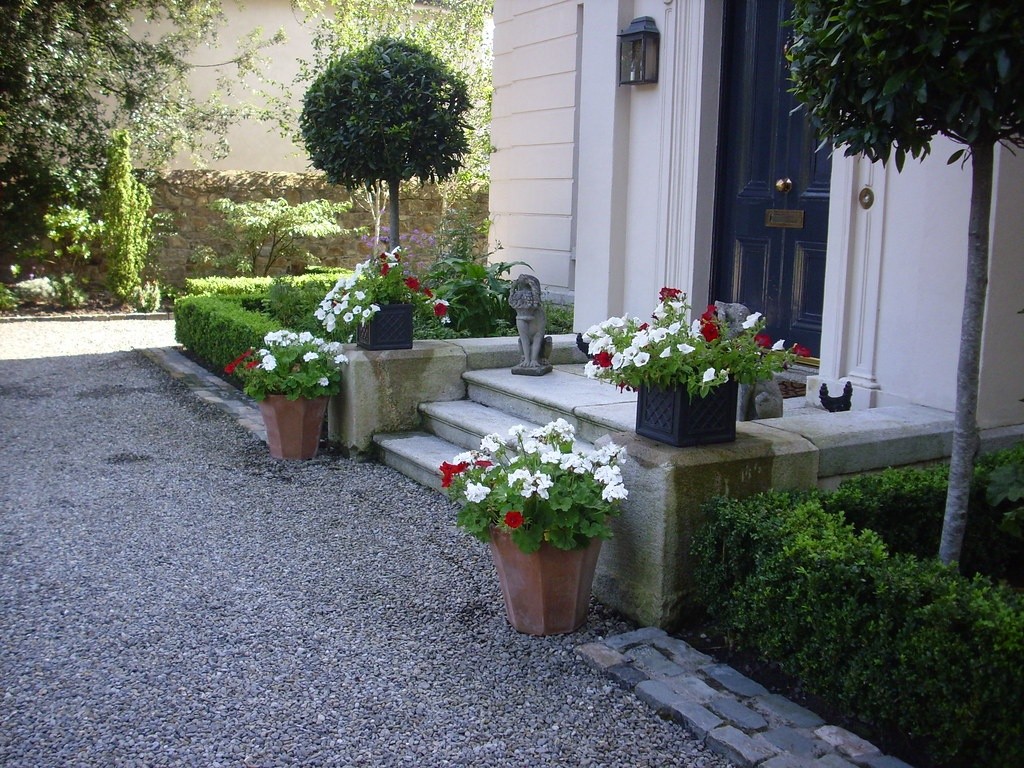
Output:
[314,246,451,333]
[576,287,810,400]
[440,418,629,554]
[224,329,349,401]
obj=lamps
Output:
[616,16,660,86]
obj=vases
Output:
[634,373,739,447]
[255,392,332,461]
[484,534,607,636]
[355,301,414,351]
